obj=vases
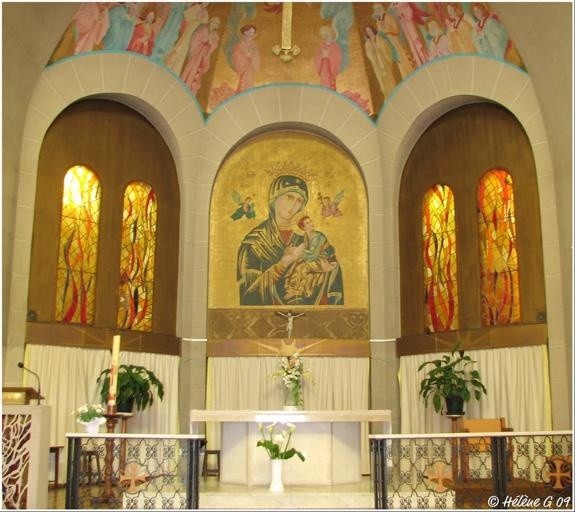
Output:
[76,416,107,433]
[269,458,285,492]
[284,379,299,405]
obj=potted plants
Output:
[417,355,486,414]
[96,365,164,412]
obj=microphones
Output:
[18,362,41,404]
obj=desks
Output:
[189,410,392,486]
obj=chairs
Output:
[460,417,514,481]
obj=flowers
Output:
[257,420,306,462]
[71,404,105,422]
[267,352,316,389]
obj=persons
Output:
[361,2,510,96]
[283,216,346,307]
[315,26,342,93]
[123,8,157,57]
[230,24,263,93]
[164,3,212,73]
[322,196,342,218]
[229,196,253,220]
[66,3,111,54]
[180,16,223,95]
[234,173,308,306]
[96,2,141,52]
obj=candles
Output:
[108,334,121,404]
